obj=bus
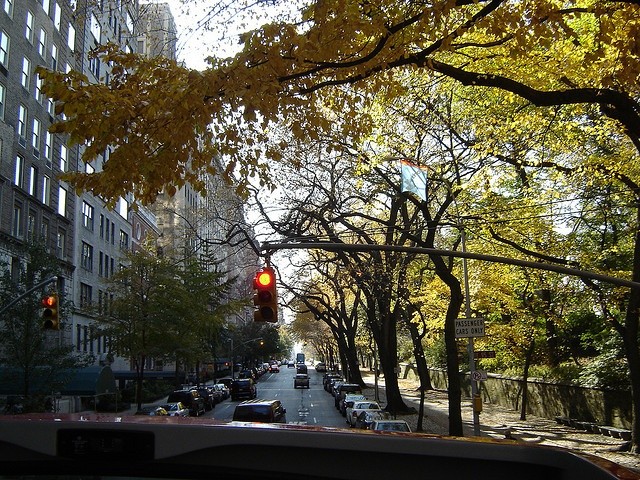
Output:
[297,353,305,364]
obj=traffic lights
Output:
[253,271,279,323]
[41,294,60,331]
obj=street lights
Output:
[328,215,383,404]
[381,155,481,439]
[231,337,263,378]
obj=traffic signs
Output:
[471,371,488,381]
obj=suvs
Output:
[238,370,256,382]
[317,363,326,372]
[345,400,382,428]
[233,399,287,423]
[167,389,206,416]
[293,374,311,389]
[217,379,234,395]
[334,384,362,410]
[207,386,223,403]
[248,367,259,380]
[188,386,215,410]
[230,379,257,401]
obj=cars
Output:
[270,364,279,373]
[366,420,412,433]
[213,384,230,400]
[338,394,368,416]
[297,364,308,373]
[354,411,394,430]
[150,402,189,417]
[324,379,344,393]
[287,361,295,368]
[323,372,342,385]
